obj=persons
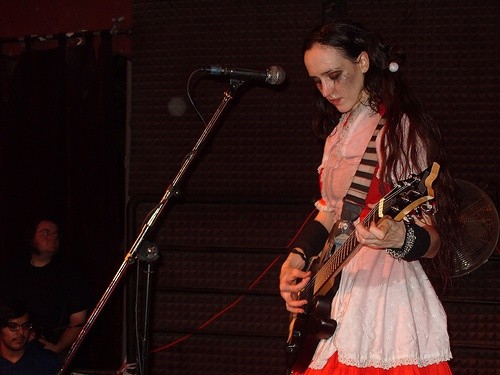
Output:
[0,216,91,375]
[0,305,59,375]
[280,22,464,375]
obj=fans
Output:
[448,179,500,278]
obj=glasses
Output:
[6,322,33,331]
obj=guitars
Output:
[282,159,442,374]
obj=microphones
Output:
[203,65,286,85]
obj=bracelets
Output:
[289,249,307,267]
[386,222,416,260]
